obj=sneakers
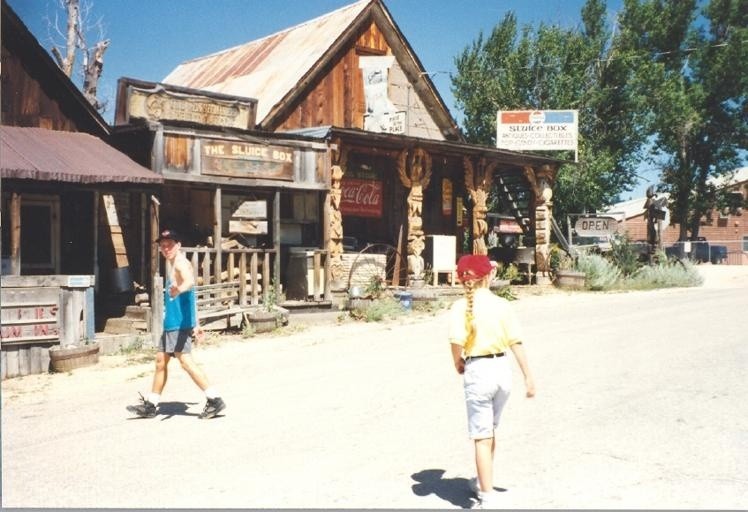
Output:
[469,477,492,501]
[200,398,225,418]
[127,401,156,417]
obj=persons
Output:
[448,253,536,511]
[125,228,226,421]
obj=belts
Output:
[471,353,503,357]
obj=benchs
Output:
[185,282,245,330]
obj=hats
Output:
[457,255,491,281]
[156,230,181,244]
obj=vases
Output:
[556,271,586,288]
